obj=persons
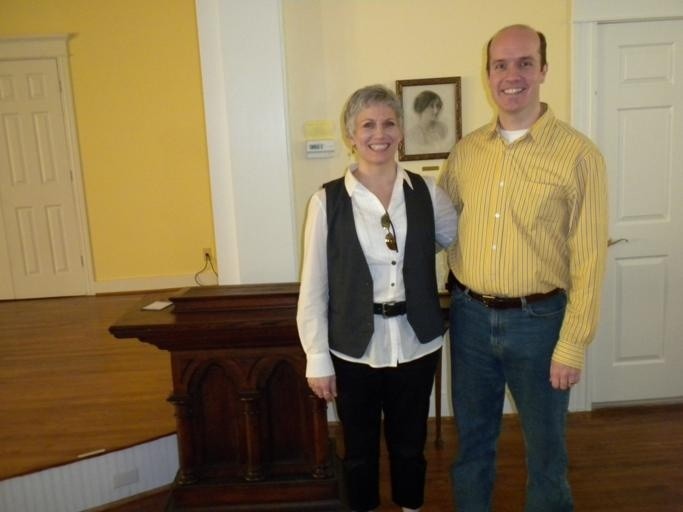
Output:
[411,91,450,145]
[297,84,459,511]
[430,25,609,512]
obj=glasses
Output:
[380,210,399,253]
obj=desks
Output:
[108,282,344,512]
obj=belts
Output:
[449,269,561,309]
[372,301,406,316]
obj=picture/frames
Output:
[396,76,462,161]
